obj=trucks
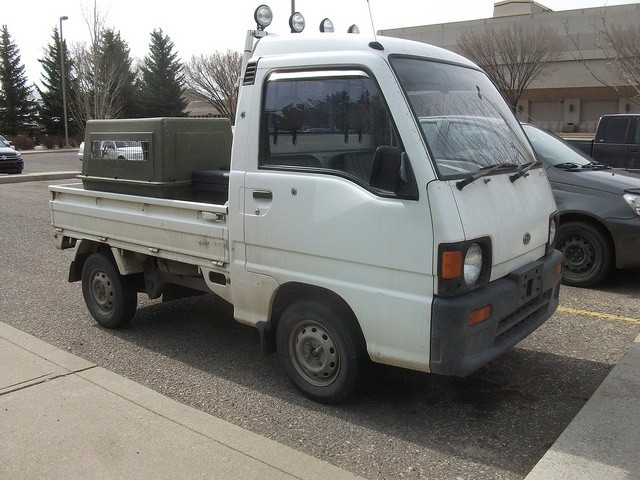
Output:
[47,4,564,404]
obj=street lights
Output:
[58,16,69,147]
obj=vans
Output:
[77,138,144,162]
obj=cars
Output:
[0,135,15,151]
[0,138,24,174]
[403,116,640,287]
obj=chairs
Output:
[370,147,405,190]
[401,152,416,182]
[262,156,322,167]
[343,153,375,182]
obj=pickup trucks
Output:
[561,114,640,170]
